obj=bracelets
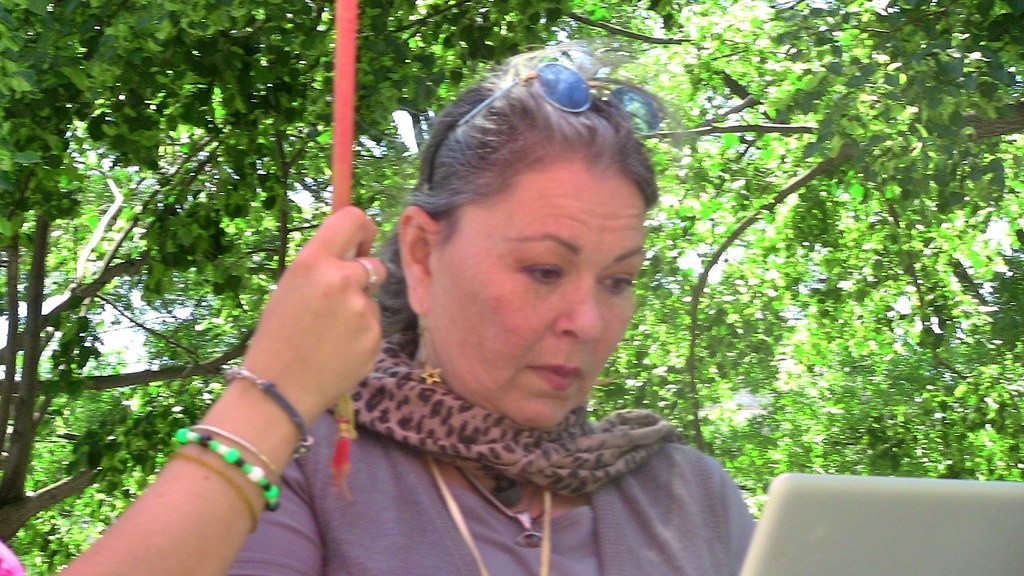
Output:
[168,424,288,533]
[220,365,313,460]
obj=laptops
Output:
[739,472,1024,576]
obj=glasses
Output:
[451,59,675,136]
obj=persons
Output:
[56,49,759,576]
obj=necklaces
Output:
[456,464,543,546]
